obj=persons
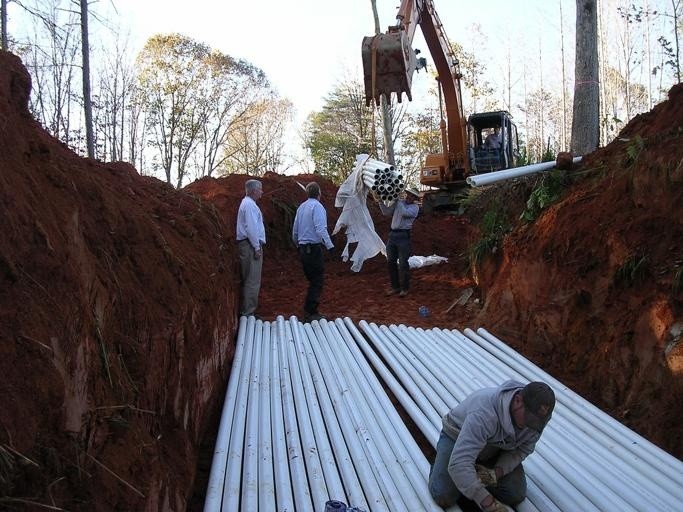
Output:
[291,181,335,324]
[428,378,556,512]
[373,187,420,298]
[479,123,510,162]
[236,179,266,323]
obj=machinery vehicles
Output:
[362,1,520,187]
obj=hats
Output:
[406,187,421,200]
[521,381,555,434]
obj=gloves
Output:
[484,499,509,512]
[474,463,497,486]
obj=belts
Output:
[389,228,410,232]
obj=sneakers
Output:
[400,291,408,296]
[387,290,400,295]
